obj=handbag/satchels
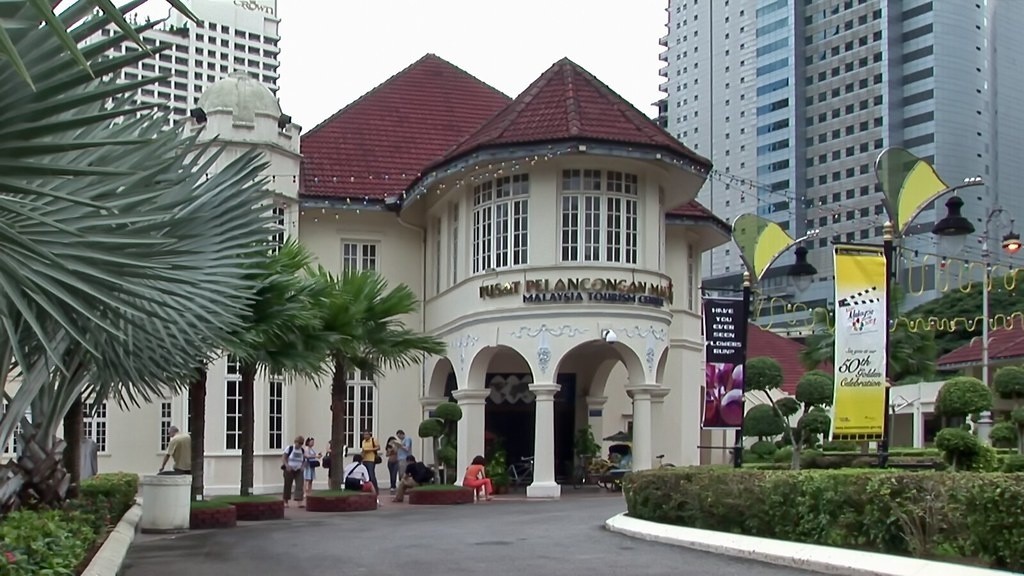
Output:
[344,478,360,490]
[323,457,331,468]
[374,456,382,463]
[309,461,320,468]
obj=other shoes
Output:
[404,489,409,495]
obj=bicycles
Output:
[603,465,625,491]
[573,449,612,489]
[505,456,534,493]
[655,454,676,469]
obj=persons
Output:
[303,437,322,493]
[392,430,412,494]
[392,454,433,503]
[157,426,192,475]
[282,436,306,508]
[346,454,380,505]
[325,438,334,488]
[385,437,399,495]
[463,456,496,502]
[361,430,381,496]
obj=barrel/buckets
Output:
[139,474,193,533]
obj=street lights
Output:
[979,207,1023,445]
[876,176,985,469]
[732,230,822,469]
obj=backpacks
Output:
[281,446,305,470]
[414,468,434,484]
